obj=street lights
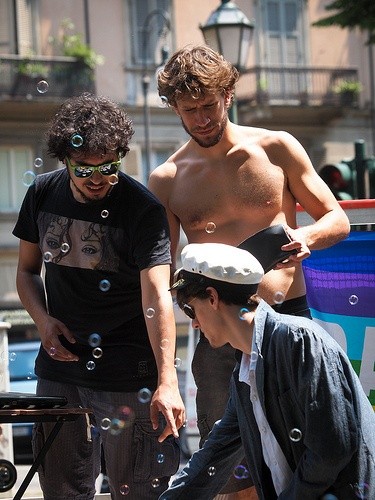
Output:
[199,0,255,126]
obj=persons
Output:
[148,47,351,500]
[157,242,375,500]
[317,163,345,203]
[11,93,187,500]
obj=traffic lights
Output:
[317,160,358,203]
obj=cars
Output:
[6,342,41,455]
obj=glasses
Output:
[65,152,122,178]
[182,296,199,319]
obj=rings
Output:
[48,348,56,356]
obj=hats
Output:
[168,243,264,295]
